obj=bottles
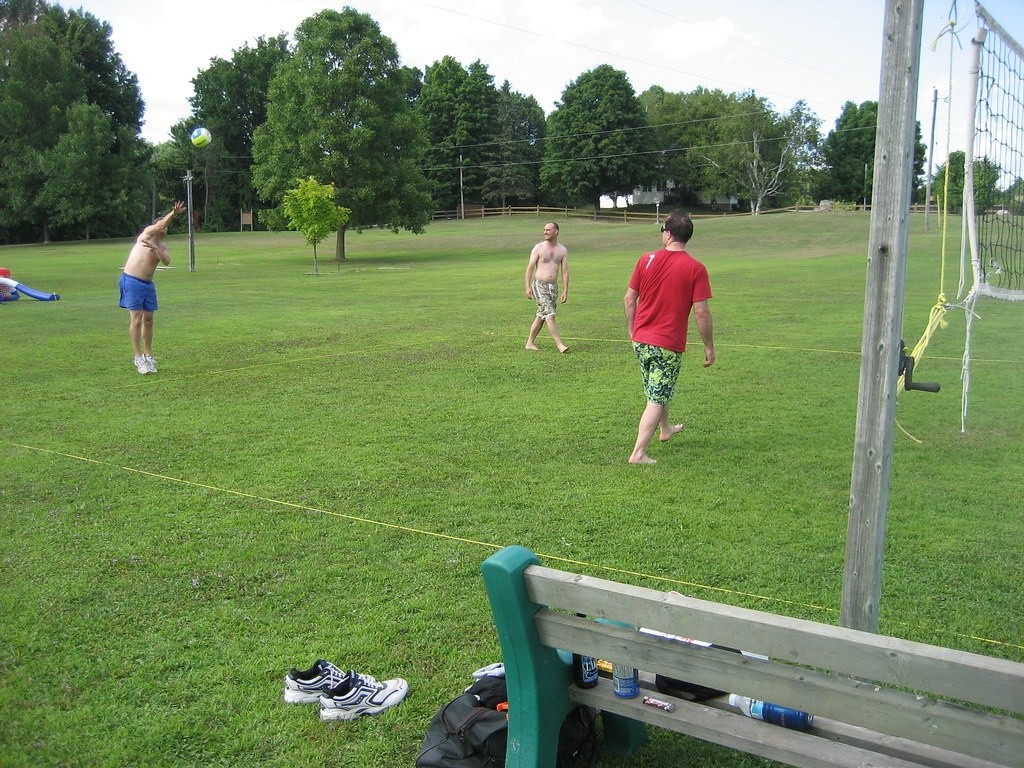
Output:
[729,693,814,732]
[572,612,598,689]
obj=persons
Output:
[119,200,187,374]
[623,210,718,465]
[524,221,570,353]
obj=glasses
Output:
[661,226,672,234]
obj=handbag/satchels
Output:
[415,683,596,768]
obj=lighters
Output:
[642,696,675,713]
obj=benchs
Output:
[479,544,1024,767]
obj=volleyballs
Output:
[190,128,212,148]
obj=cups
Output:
[612,663,640,699]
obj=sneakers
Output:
[143,354,158,372]
[319,670,408,720]
[284,659,347,703]
[134,356,149,374]
